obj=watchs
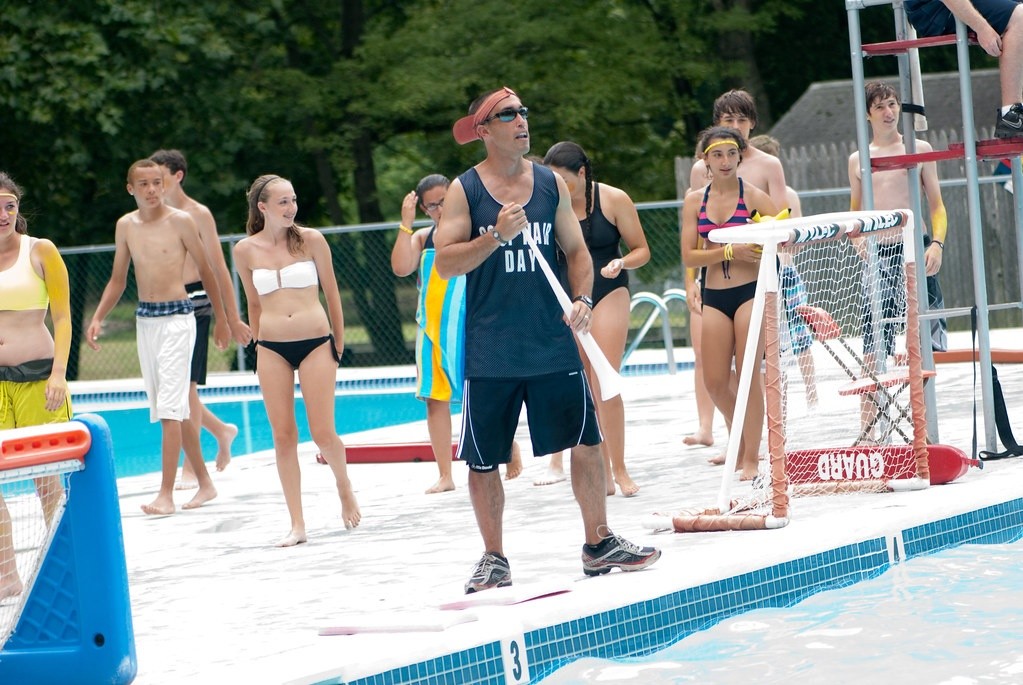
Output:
[489,227,508,244]
[930,240,945,251]
[573,295,593,310]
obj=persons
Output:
[148,147,253,492]
[683,87,818,463]
[432,82,663,595]
[848,79,949,448]
[390,174,523,494]
[903,0,1023,139]
[524,141,652,497]
[681,123,783,480]
[0,169,72,608]
[231,172,363,548]
[84,160,230,517]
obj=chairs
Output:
[792,304,937,448]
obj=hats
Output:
[453,84,517,144]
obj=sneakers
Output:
[466,554,511,593]
[582,523,661,576]
[993,106,1023,139]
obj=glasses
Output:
[424,199,444,211]
[488,106,529,123]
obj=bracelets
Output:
[724,243,734,261]
[399,223,415,236]
[336,350,343,355]
[615,259,624,269]
